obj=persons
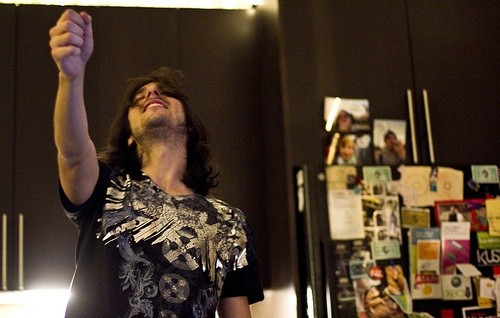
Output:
[334,111,353,133]
[336,134,361,165]
[448,206,457,222]
[49,8,266,318]
[428,167,438,193]
[374,130,406,165]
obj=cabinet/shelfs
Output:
[0,3,180,298]
[277,1,500,318]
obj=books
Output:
[325,94,500,318]
